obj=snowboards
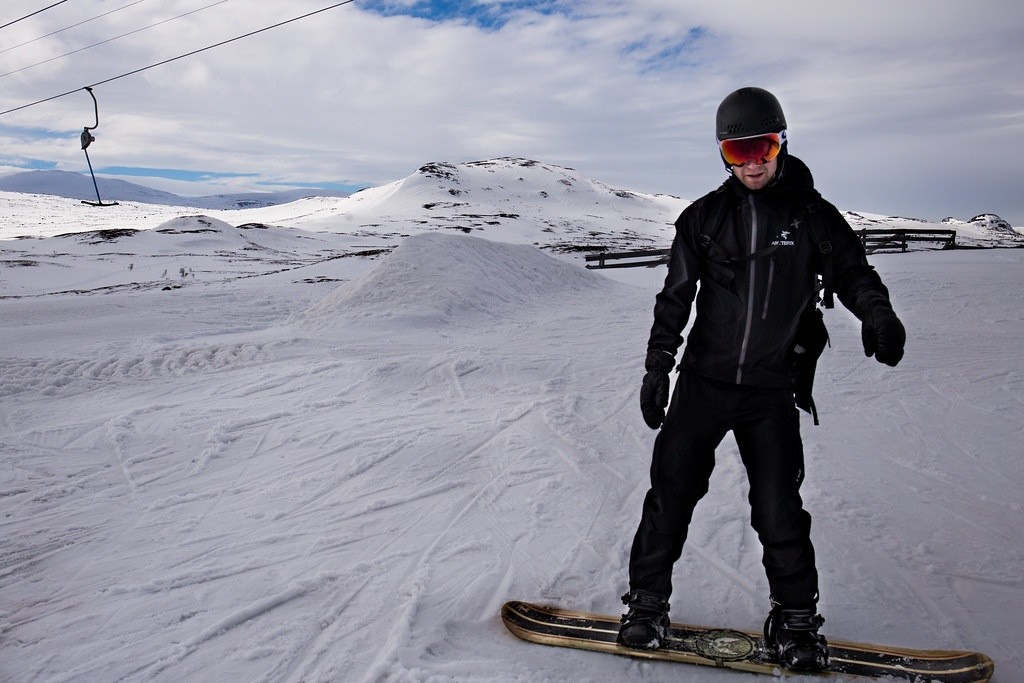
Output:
[499,601,997,683]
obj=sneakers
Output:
[618,586,671,652]
[763,608,831,676]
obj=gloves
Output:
[640,350,676,430]
[854,290,907,367]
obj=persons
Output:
[616,87,908,673]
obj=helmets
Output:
[716,86,787,143]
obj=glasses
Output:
[720,130,786,167]
